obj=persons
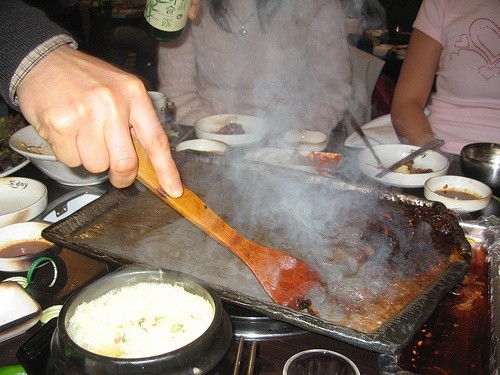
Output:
[158,0,354,132]
[390,0,500,155]
[0,0,184,198]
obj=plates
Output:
[0,158,31,178]
[242,147,343,175]
[0,177,48,228]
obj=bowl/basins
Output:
[175,139,230,153]
[8,125,109,186]
[282,349,360,375]
[459,142,500,194]
[193,113,267,147]
[0,221,62,272]
[424,175,492,212]
[276,129,329,151]
[357,144,450,188]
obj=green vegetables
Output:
[121,321,142,343]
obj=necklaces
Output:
[229,3,256,37]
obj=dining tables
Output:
[0,135,500,375]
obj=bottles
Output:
[141,0,191,42]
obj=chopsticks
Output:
[371,138,445,178]
[233,335,257,375]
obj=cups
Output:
[147,91,166,127]
[345,18,362,32]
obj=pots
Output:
[50,263,233,375]
